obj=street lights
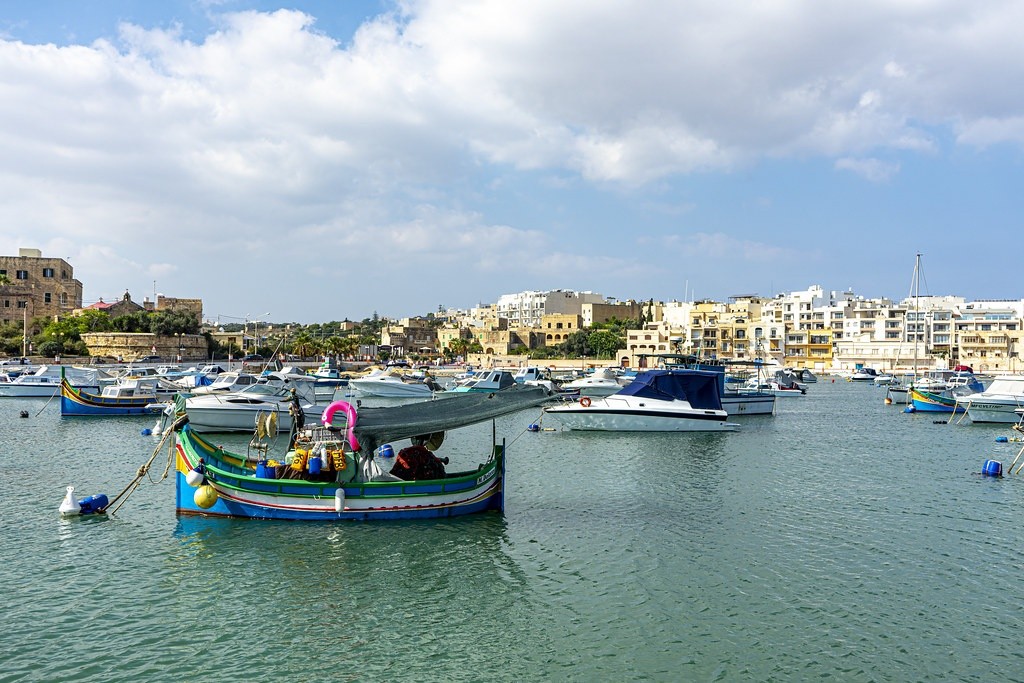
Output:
[278,334,288,355]
[51,332,65,365]
[23,301,29,357]
[174,332,186,364]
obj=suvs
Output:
[2,357,32,366]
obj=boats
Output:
[542,366,745,435]
[0,305,818,434]
[952,374,1024,423]
[849,249,984,413]
[165,389,508,522]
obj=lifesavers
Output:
[579,396,592,407]
